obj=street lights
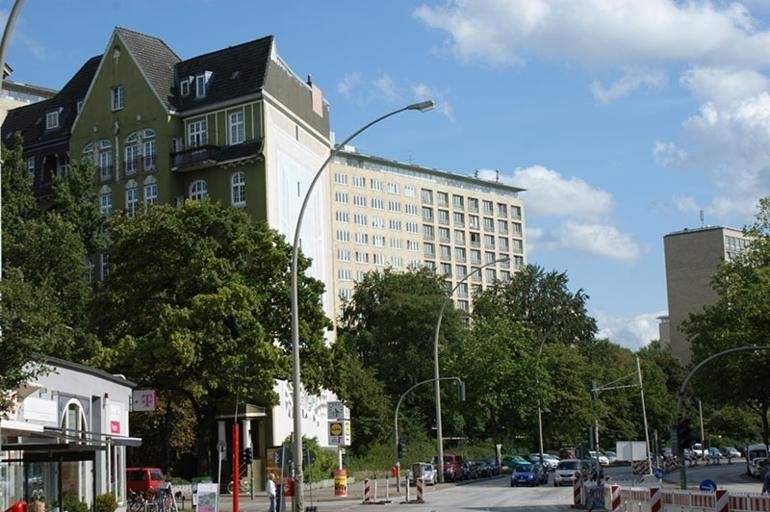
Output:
[535,309,578,465]
[291,99,436,512]
[434,256,512,483]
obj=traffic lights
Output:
[244,447,253,465]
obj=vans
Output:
[126,467,166,501]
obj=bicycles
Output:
[226,474,255,495]
[125,487,171,512]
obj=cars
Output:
[27,472,43,490]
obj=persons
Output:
[761,466,770,495]
[267,473,278,512]
